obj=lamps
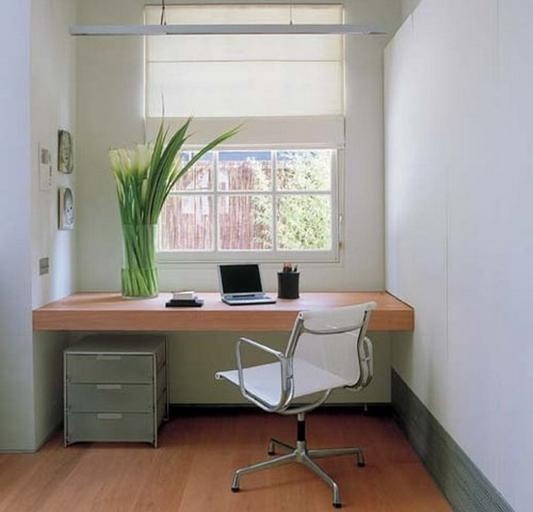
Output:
[67,1,392,39]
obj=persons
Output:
[60,134,73,169]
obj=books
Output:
[164,291,204,308]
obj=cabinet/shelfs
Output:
[60,333,167,447]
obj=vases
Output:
[119,224,157,297]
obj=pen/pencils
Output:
[283,263,298,273]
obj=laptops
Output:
[217,262,276,305]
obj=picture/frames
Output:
[57,128,75,175]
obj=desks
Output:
[33,290,414,413]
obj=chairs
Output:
[213,299,374,507]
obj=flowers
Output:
[107,96,247,298]
